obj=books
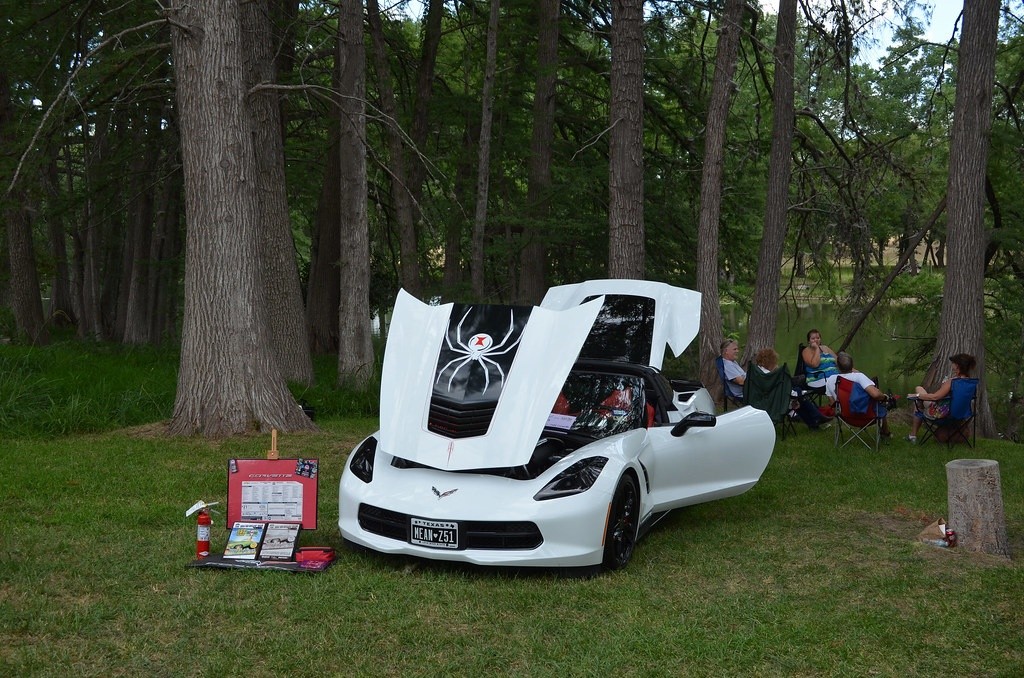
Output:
[222,522,302,562]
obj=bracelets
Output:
[917,392,921,398]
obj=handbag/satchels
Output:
[818,404,834,417]
[916,517,947,540]
[936,419,969,443]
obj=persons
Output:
[825,353,894,445]
[721,339,833,429]
[802,329,860,405]
[905,353,976,445]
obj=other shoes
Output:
[809,415,833,431]
[791,374,805,386]
[881,432,893,445]
[903,433,917,442]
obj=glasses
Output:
[724,340,733,348]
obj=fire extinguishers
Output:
[197,502,221,560]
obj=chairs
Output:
[830,375,888,453]
[742,360,798,441]
[597,386,655,430]
[907,377,979,450]
[551,390,571,415]
[715,356,744,413]
[794,343,827,408]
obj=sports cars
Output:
[338,277,776,576]
